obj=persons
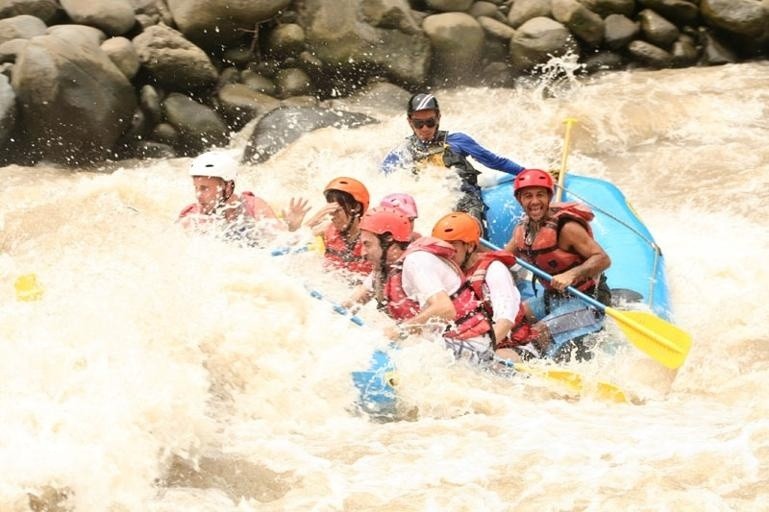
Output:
[173,150,310,231]
[432,212,545,370]
[381,93,560,241]
[288,177,375,276]
[340,206,492,368]
[478,169,611,351]
[373,190,425,241]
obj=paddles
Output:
[478,237,693,369]
[448,335,626,403]
[10,238,325,303]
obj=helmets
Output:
[406,93,440,113]
[190,152,238,197]
[323,177,481,249]
[514,170,553,197]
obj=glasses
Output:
[408,117,438,129]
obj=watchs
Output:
[396,325,410,340]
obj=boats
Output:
[359,167,678,428]
